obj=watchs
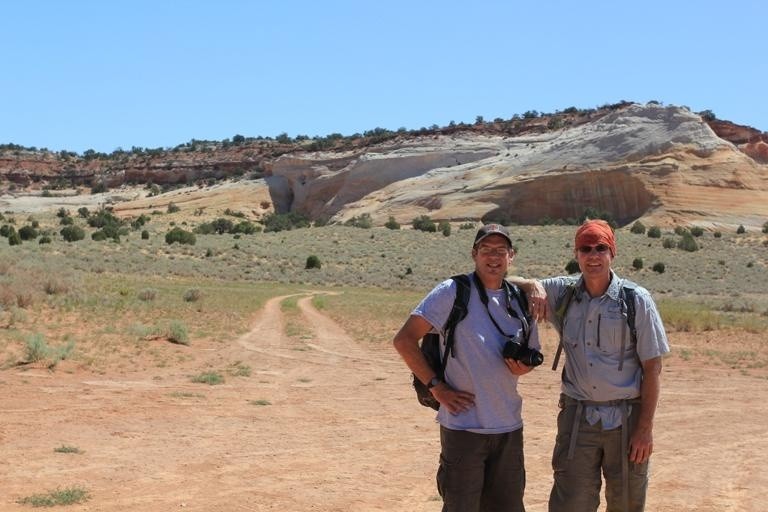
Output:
[426,375,442,391]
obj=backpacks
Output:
[412,274,531,411]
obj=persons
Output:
[507,219,669,512]
[394,220,540,512]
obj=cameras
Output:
[503,341,543,367]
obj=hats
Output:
[575,221,616,257]
[474,224,512,246]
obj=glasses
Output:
[580,244,609,252]
[474,247,511,254]
